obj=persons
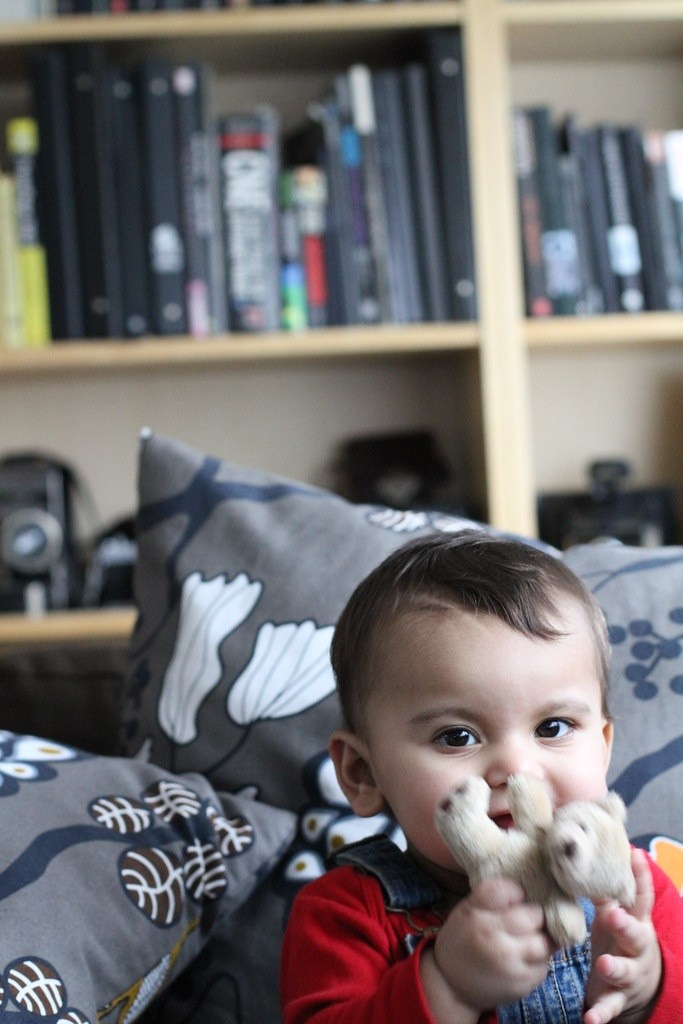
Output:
[283,530,683,1024]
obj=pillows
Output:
[2,422,683,1024]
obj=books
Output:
[0,0,683,342]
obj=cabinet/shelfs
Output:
[1,0,683,647]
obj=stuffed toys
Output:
[435,771,637,946]
